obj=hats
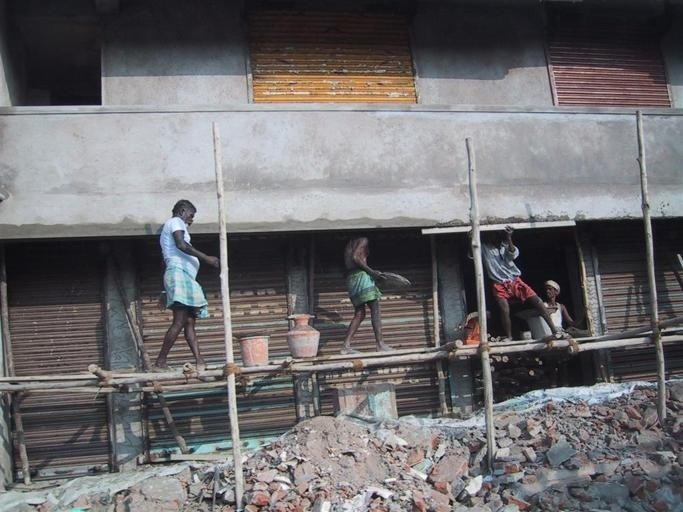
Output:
[544,280,560,296]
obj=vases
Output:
[284,312,322,359]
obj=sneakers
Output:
[554,328,570,339]
[503,336,512,342]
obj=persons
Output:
[152,200,220,374]
[540,278,586,337]
[339,230,396,353]
[468,224,559,341]
